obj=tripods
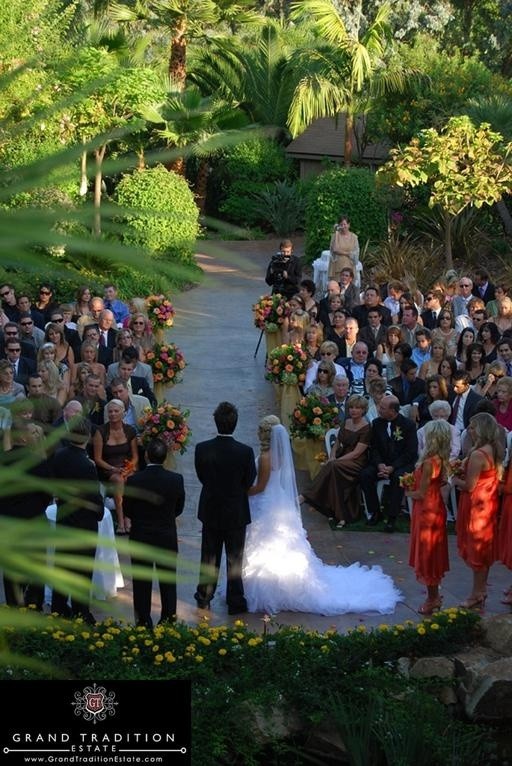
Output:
[254,275,292,358]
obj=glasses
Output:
[1,290,63,352]
[424,297,433,302]
[319,351,332,356]
[132,321,143,325]
[438,316,451,320]
[459,284,470,288]
[356,351,368,355]
[318,369,328,374]
[473,317,481,320]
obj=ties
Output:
[387,422,392,438]
[452,394,460,426]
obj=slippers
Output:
[116,526,131,536]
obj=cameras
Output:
[336,224,344,231]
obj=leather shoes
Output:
[384,520,394,532]
[366,512,384,526]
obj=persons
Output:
[0,215,512,629]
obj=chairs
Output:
[323,421,511,525]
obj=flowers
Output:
[135,400,192,454]
[250,292,292,336]
[143,342,188,383]
[144,294,176,335]
[265,342,310,388]
[287,392,342,442]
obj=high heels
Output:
[420,596,440,614]
[501,588,511,604]
[460,591,487,609]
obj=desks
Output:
[312,250,363,289]
[42,501,126,610]
